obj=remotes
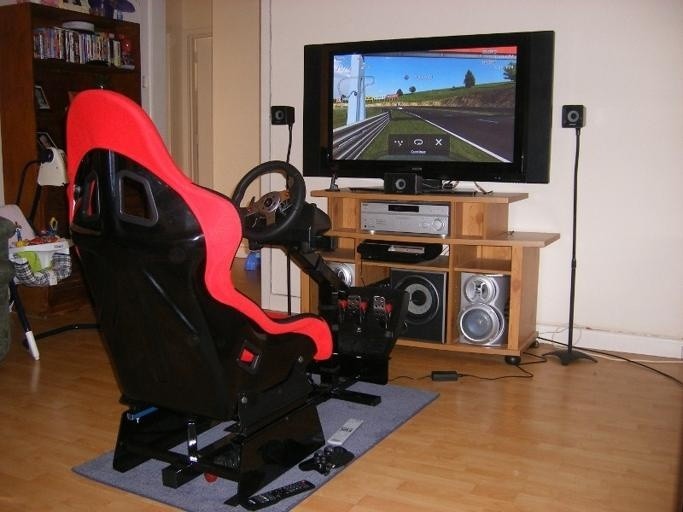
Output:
[244,479,316,511]
[326,417,366,447]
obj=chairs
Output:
[65,89,327,499]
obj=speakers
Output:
[380,174,421,195]
[386,269,449,345]
[561,103,587,129]
[271,105,295,127]
[457,274,508,347]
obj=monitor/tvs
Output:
[304,29,555,194]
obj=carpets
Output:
[70,375,439,512]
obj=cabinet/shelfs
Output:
[295,187,560,363]
[0,0,142,319]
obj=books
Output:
[33,21,127,66]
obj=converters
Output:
[430,369,458,381]
